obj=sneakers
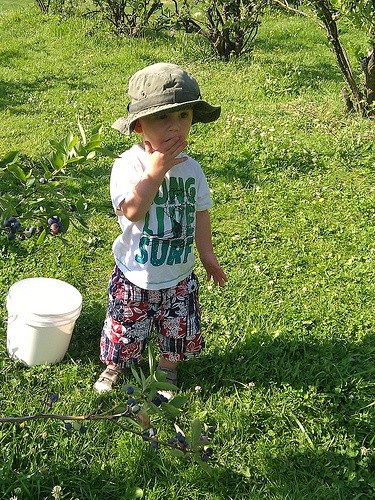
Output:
[92,365,122,394]
[152,369,177,400]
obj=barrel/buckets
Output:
[6,277,82,368]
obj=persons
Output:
[90,62,230,402]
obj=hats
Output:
[111,63,221,135]
